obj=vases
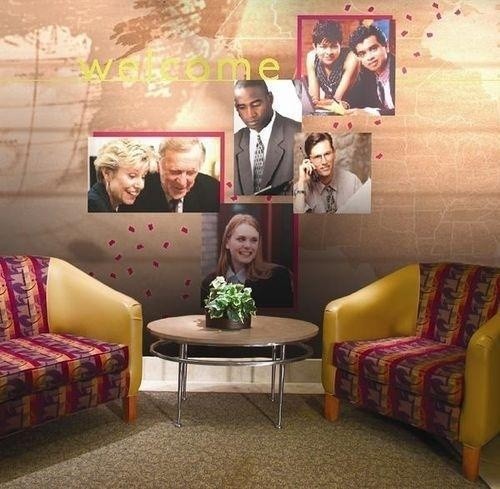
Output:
[206,312,251,331]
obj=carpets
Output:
[0,391,490,489]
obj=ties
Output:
[169,199,180,214]
[253,136,265,193]
[229,276,238,284]
[324,186,338,215]
[378,81,385,104]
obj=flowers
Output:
[204,275,256,325]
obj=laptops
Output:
[302,76,336,114]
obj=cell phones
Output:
[299,147,320,182]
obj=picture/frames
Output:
[296,14,397,116]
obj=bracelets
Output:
[293,187,305,196]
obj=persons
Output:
[293,132,364,214]
[118,136,220,212]
[87,139,151,213]
[341,24,395,111]
[200,213,295,308]
[232,80,301,197]
[305,19,359,108]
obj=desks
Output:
[147,315,319,428]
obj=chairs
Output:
[322,260,500,480]
[0,254,143,441]
